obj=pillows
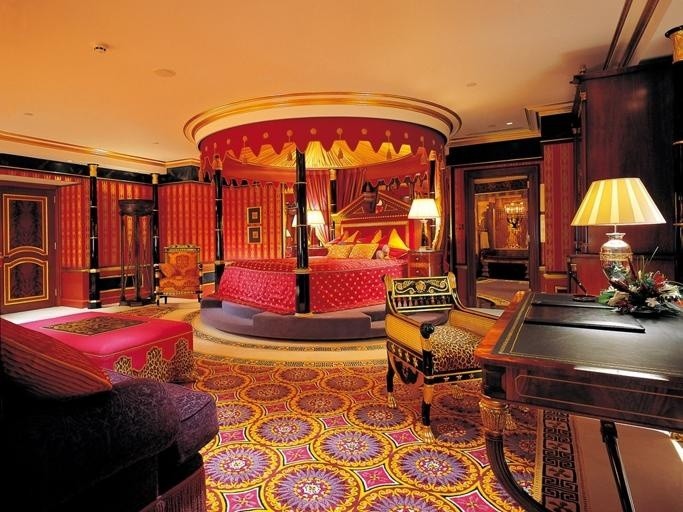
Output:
[327,245,352,258]
[0,318,113,397]
[349,243,379,259]
[377,246,406,259]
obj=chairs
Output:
[379,271,517,443]
[152,244,202,306]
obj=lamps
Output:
[505,207,523,228]
[569,176,666,289]
[407,199,437,250]
[306,211,325,248]
[290,215,296,227]
[285,230,291,237]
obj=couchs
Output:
[0,317,219,511]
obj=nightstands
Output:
[307,247,327,256]
[406,251,441,277]
[292,245,297,256]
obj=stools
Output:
[18,311,194,382]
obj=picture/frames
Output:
[246,206,261,224]
[246,226,262,244]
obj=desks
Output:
[473,287,683,511]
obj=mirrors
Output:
[282,192,309,259]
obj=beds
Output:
[216,190,421,313]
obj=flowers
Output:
[597,247,682,314]
